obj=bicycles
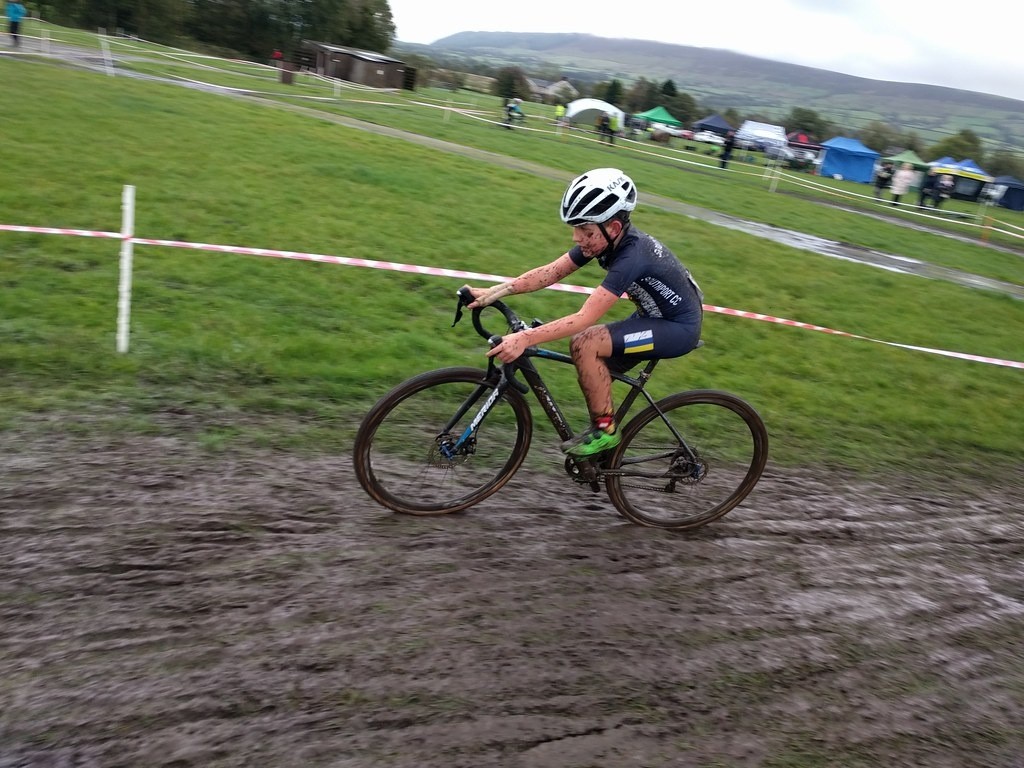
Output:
[487,114,530,135]
[351,287,771,535]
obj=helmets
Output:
[559,167,638,227]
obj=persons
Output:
[554,103,565,120]
[4,1,26,47]
[460,168,703,455]
[268,48,282,66]
[716,131,735,169]
[873,162,955,212]
[500,98,527,129]
[597,112,619,147]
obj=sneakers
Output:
[560,424,623,457]
